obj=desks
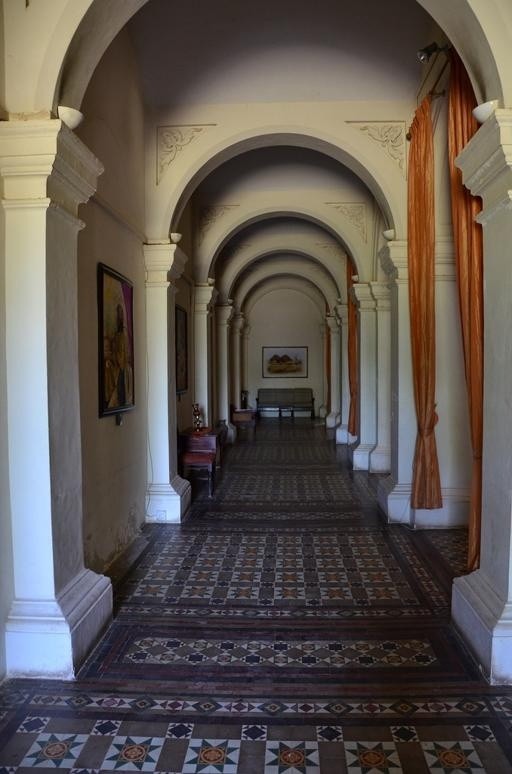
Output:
[181,427,224,470]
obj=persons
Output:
[104,300,134,407]
[102,333,117,405]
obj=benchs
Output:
[255,388,315,423]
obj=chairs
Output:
[177,425,216,498]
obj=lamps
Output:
[416,40,453,64]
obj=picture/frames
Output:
[98,263,134,427]
[175,304,188,395]
[262,346,308,378]
